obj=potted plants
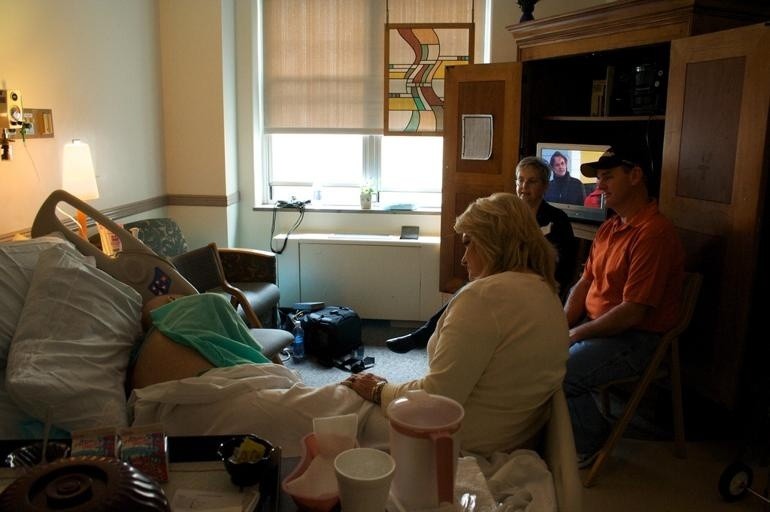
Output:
[360,186,373,209]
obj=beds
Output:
[0,232,583,512]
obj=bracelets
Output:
[371,381,385,405]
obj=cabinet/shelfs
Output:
[439,0,770,411]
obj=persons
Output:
[564,146,686,469]
[339,192,571,462]
[386,156,578,354]
[544,151,587,206]
[126,293,215,396]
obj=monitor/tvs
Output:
[536,142,612,224]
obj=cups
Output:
[386,388,467,512]
[359,193,372,209]
[334,445,396,512]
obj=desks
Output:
[273,233,443,321]
[0,435,501,512]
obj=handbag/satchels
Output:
[298,306,374,375]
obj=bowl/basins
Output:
[4,442,71,467]
[218,433,282,487]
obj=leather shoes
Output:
[385,334,415,355]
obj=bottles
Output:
[291,320,306,360]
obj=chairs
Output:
[75,217,280,328]
[583,227,709,488]
[167,243,294,366]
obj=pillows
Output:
[5,244,143,433]
[0,232,83,371]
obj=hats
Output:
[580,145,635,178]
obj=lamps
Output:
[61,140,100,243]
